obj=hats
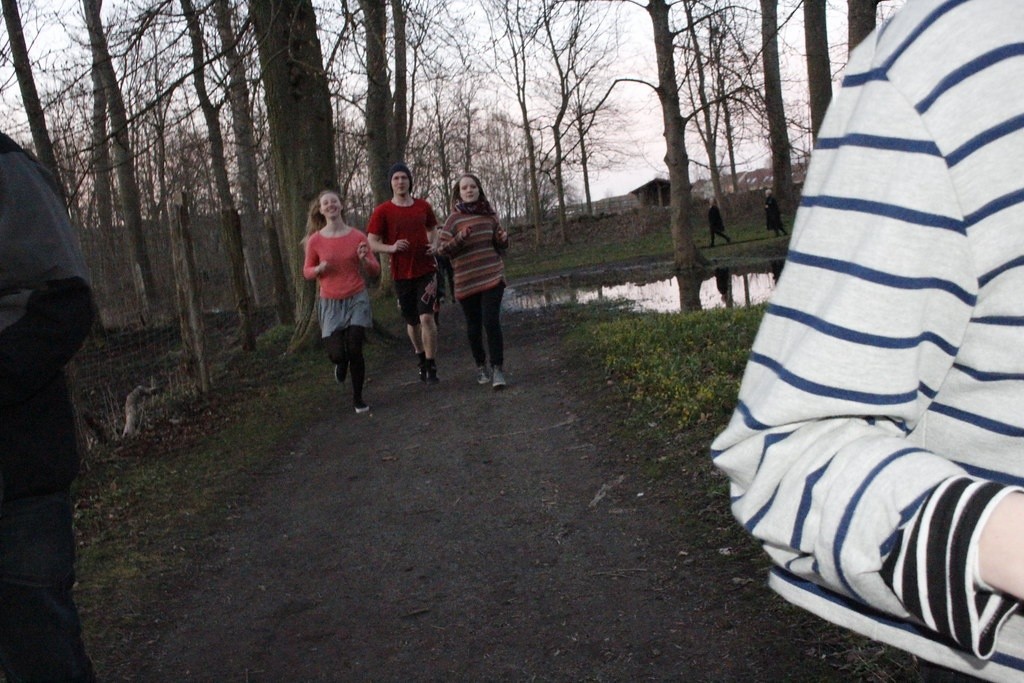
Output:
[390,164,413,195]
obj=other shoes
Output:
[335,362,349,384]
[440,296,445,304]
[452,296,456,304]
[352,398,370,413]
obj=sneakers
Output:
[417,360,439,385]
[477,365,506,390]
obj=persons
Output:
[435,226,456,304]
[707,197,730,247]
[764,189,788,237]
[707,0,1024,683]
[366,164,439,384]
[300,190,380,412]
[0,132,95,682]
[434,173,509,389]
